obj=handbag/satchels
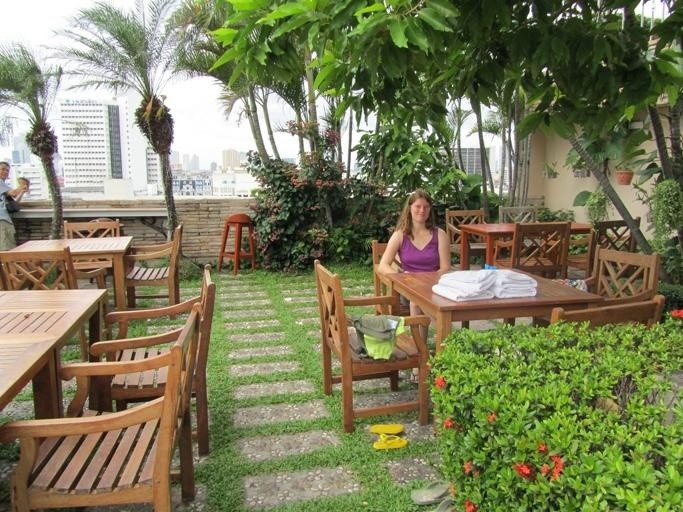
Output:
[5,195,20,213]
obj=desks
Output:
[8,234,138,310]
[1,287,108,443]
[383,266,605,356]
[457,220,611,278]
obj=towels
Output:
[429,270,540,304]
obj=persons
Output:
[0,161,28,255]
[377,190,451,383]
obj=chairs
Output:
[311,258,432,434]
[444,205,495,267]
[496,202,550,260]
[55,216,124,288]
[542,293,666,332]
[123,220,187,312]
[87,263,218,457]
[533,244,669,331]
[0,245,111,360]
[0,299,207,512]
[570,210,641,283]
[496,213,567,281]
[369,235,410,318]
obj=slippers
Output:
[409,372,419,384]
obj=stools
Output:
[214,209,257,278]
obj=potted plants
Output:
[614,159,635,185]
